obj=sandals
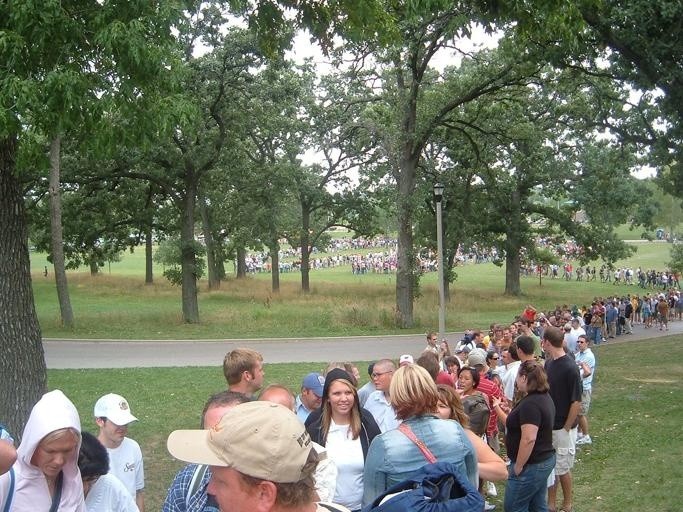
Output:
[549,507,575,512]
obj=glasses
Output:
[370,371,391,378]
[489,356,499,360]
[576,341,586,343]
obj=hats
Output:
[399,354,414,365]
[93,392,139,426]
[302,372,326,398]
[165,401,313,482]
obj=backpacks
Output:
[361,461,484,512]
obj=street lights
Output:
[432,181,447,343]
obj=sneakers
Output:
[484,501,495,511]
[576,433,592,444]
[486,481,497,496]
[601,321,671,340]
